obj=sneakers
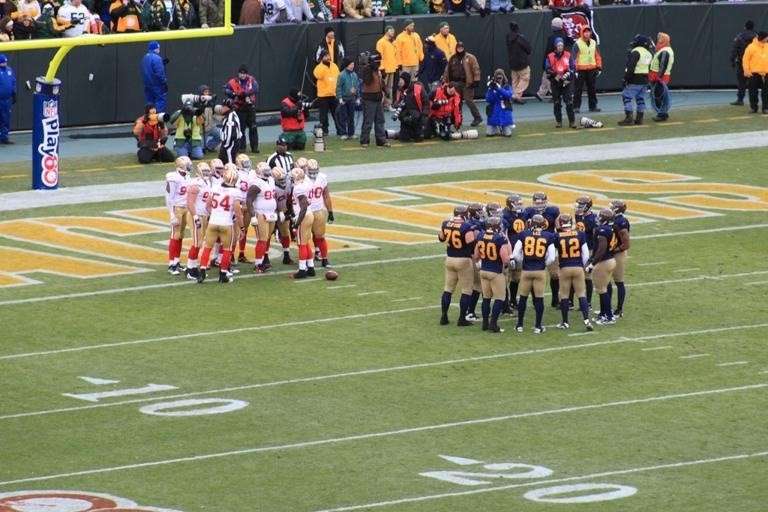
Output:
[340,135,391,148]
[568,121,578,128]
[470,118,482,126]
[439,298,624,334]
[555,121,562,127]
[167,253,332,283]
[574,106,603,111]
[251,148,260,153]
[652,111,668,121]
[239,149,245,152]
[729,100,767,115]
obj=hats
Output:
[384,18,450,31]
[321,28,335,55]
[147,41,162,51]
[276,139,287,145]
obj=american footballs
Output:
[325,271,338,280]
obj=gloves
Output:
[194,215,202,228]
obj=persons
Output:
[131,41,309,159]
[1,54,19,144]
[1,1,225,42]
[163,138,335,283]
[232,2,674,147]
[728,19,766,114]
[438,190,631,332]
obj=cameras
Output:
[560,68,574,83]
[298,100,312,112]
[356,4,364,9]
[391,100,403,121]
[239,92,253,107]
[435,98,448,106]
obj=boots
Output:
[635,110,645,125]
[617,110,634,126]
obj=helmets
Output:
[176,155,320,187]
[454,192,627,228]
